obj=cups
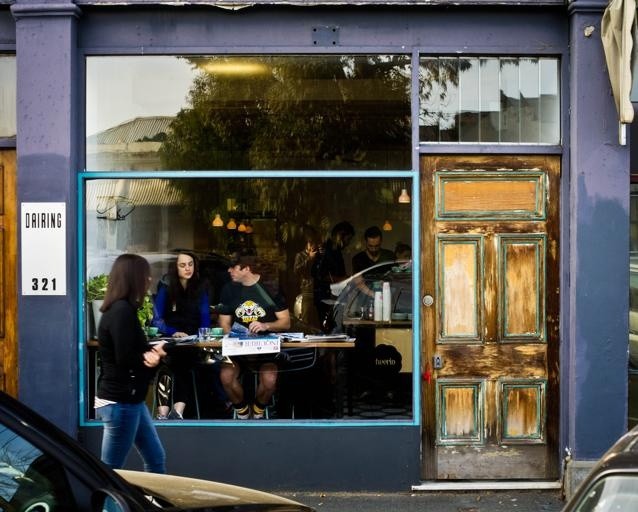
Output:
[368,280,393,323]
[198,327,224,341]
[360,306,369,320]
[141,327,159,336]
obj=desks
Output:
[86,333,357,420]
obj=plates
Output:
[391,312,412,320]
[347,318,362,321]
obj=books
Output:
[154,321,356,357]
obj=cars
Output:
[0,392,314,510]
[627,247,638,375]
[84,244,412,395]
[559,425,638,510]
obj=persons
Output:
[214,250,294,420]
[350,226,395,309]
[290,227,338,332]
[147,248,211,420]
[308,221,356,334]
[88,251,171,475]
[392,239,413,262]
